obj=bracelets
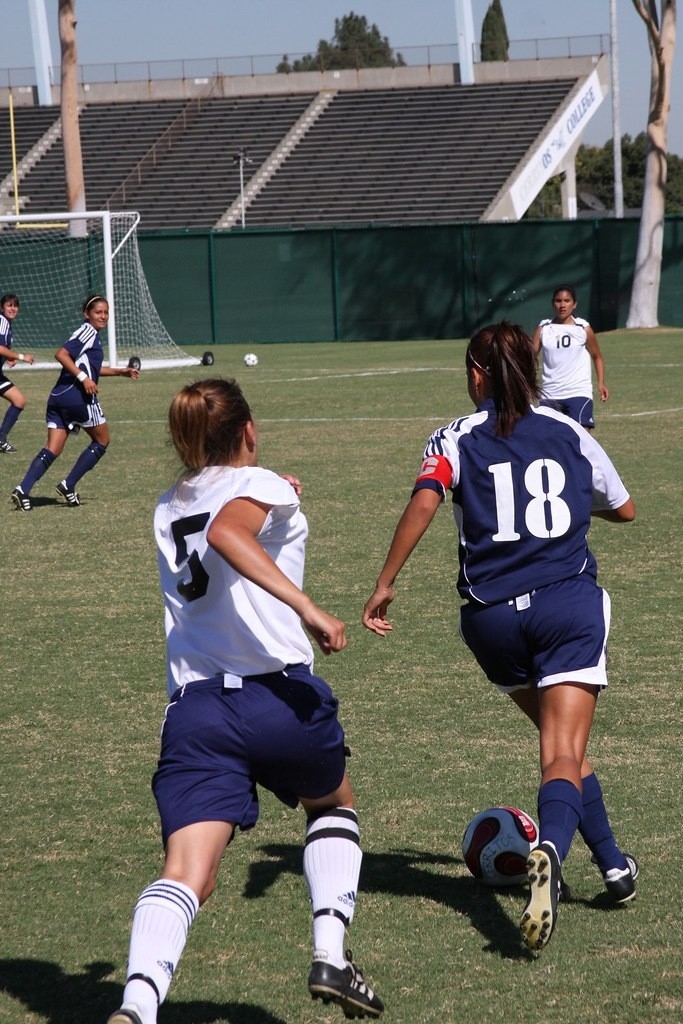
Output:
[77,371,88,382]
[19,353,24,361]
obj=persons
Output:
[362,322,637,952]
[112,380,384,1024]
[532,286,611,434]
[0,293,35,453]
[11,295,140,511]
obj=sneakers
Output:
[0,441,18,454]
[106,1002,145,1024]
[55,479,80,507]
[520,839,563,958]
[601,853,639,904]
[12,485,33,512]
[308,948,384,1020]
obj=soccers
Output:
[464,805,541,883]
[243,353,258,367]
